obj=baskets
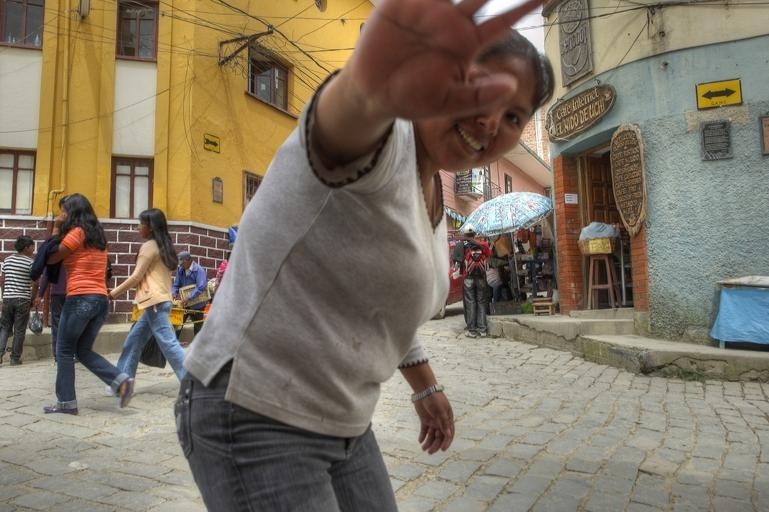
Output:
[575,234,617,258]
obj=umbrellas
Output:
[458,192,554,302]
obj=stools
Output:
[585,255,623,309]
[533,300,556,315]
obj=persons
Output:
[450,224,535,339]
[172,0,555,512]
[172,252,207,337]
[32,220,81,366]
[44,195,134,412]
[0,236,40,365]
[214,250,232,295]
[107,208,187,398]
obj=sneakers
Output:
[0,355,22,367]
[101,378,138,408]
[464,329,487,338]
[42,403,79,417]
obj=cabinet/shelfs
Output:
[612,238,634,306]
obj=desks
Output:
[713,275,769,350]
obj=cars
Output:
[427,231,495,323]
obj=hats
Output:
[463,222,475,234]
[175,250,191,265]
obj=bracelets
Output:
[409,380,447,402]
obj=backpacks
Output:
[461,239,489,279]
